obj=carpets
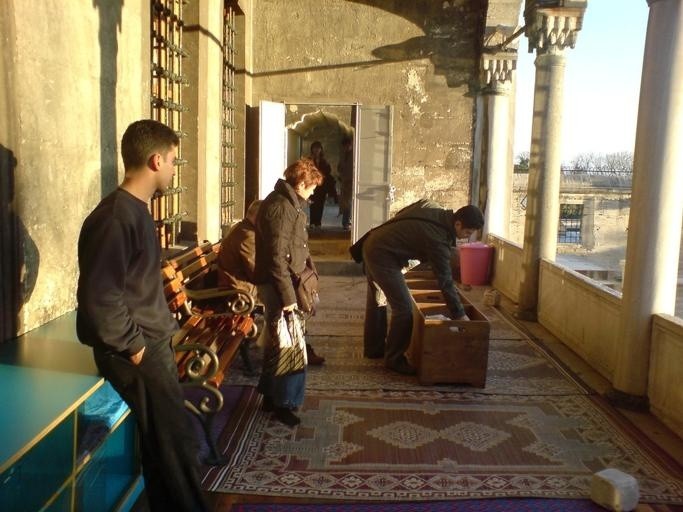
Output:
[200,275,683,512]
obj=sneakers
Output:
[305,344,325,364]
[308,220,352,232]
[386,357,417,376]
[262,397,300,425]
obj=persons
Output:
[217,199,325,366]
[361,198,484,375]
[308,141,331,229]
[254,158,324,426]
[77,119,207,511]
[338,140,353,230]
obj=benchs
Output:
[161,241,258,415]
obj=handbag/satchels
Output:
[347,226,368,264]
[269,307,308,378]
[296,267,321,318]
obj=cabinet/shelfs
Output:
[0,364,144,512]
